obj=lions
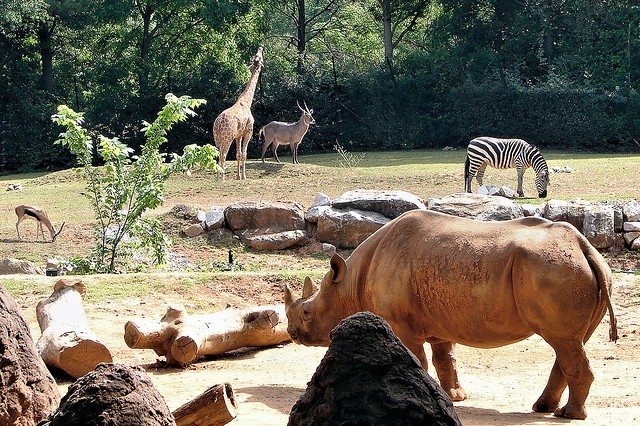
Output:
[464,136,550,198]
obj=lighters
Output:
[212,46,264,181]
